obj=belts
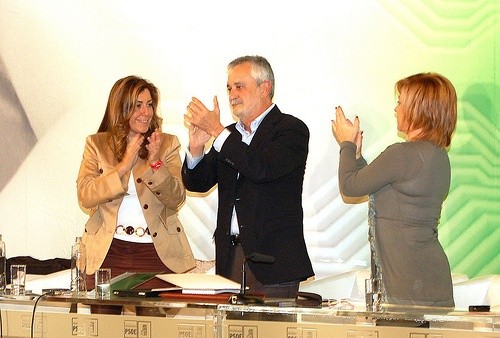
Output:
[231,234,242,246]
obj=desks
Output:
[0,291,500,338]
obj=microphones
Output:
[227,253,275,305]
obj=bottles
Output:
[0,234,6,296]
[70,237,86,296]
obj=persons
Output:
[180,55,315,322]
[331,72,457,329]
[75,74,197,318]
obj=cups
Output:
[365,279,382,311]
[10,265,26,296]
[95,269,111,299]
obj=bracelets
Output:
[152,160,162,170]
